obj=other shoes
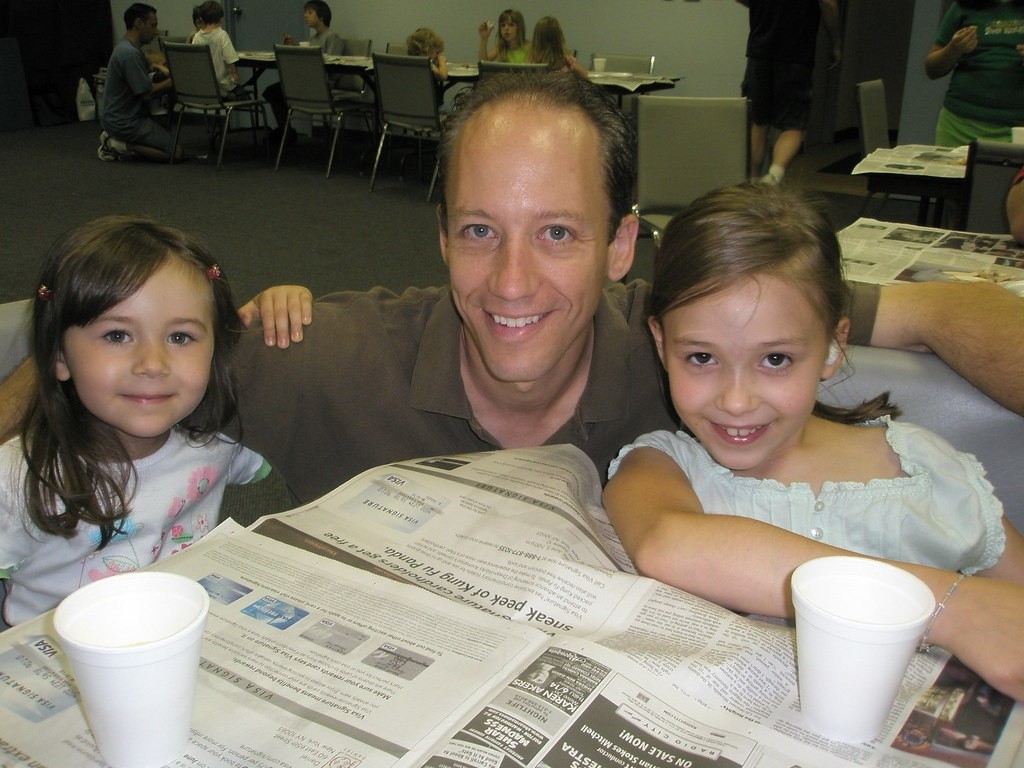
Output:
[761,174,785,189]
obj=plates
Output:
[587,72,608,77]
[608,72,632,77]
[239,52,255,57]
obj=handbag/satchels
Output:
[77,78,95,121]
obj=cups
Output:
[594,57,606,71]
[1012,127,1024,145]
[791,556,935,742]
[299,42,309,46]
[53,571,211,768]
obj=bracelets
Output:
[917,573,964,651]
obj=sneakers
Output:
[97,130,115,162]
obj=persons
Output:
[186,4,201,43]
[527,14,589,77]
[1006,166,1024,245]
[478,8,532,63]
[604,182,1024,699]
[260,0,344,147]
[175,61,1024,529]
[925,0,1024,149]
[97,3,178,161]
[406,27,453,114]
[736,0,840,189]
[0,216,314,633]
[191,0,250,99]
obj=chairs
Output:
[386,43,409,57]
[328,40,373,95]
[626,96,752,251]
[590,56,658,110]
[365,53,456,203]
[854,78,973,228]
[163,42,269,168]
[267,45,371,179]
[477,61,548,98]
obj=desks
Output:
[231,50,675,150]
